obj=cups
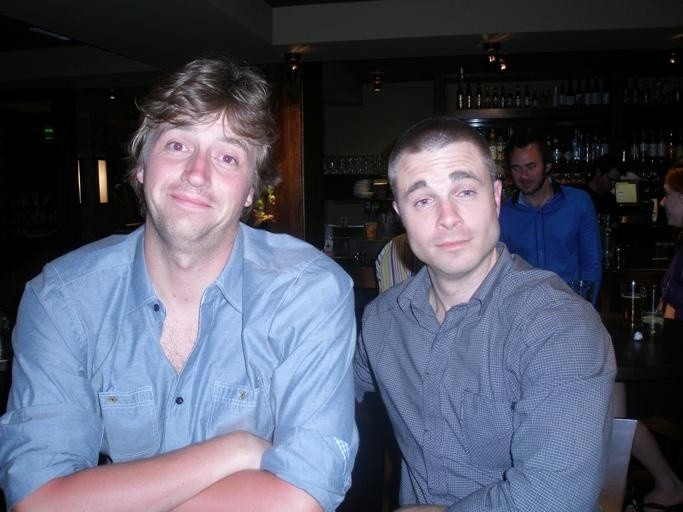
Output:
[621,279,668,341]
[365,221,379,238]
[567,278,594,304]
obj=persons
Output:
[0,54,360,512]
[352,115,619,512]
[611,166,683,512]
[499,130,603,311]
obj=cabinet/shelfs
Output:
[320,74,683,268]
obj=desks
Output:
[592,308,682,381]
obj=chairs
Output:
[600,270,667,319]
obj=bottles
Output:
[453,76,683,198]
